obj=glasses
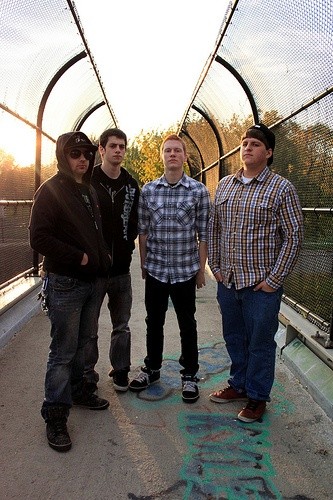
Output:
[64,149,94,160]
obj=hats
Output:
[63,132,98,151]
[248,123,275,164]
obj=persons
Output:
[84,129,140,392]
[29,131,110,453]
[207,123,304,423]
[129,135,211,403]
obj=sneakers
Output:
[209,385,248,402]
[129,366,160,389]
[237,400,266,422]
[179,374,199,400]
[72,392,109,408]
[112,370,129,390]
[46,421,72,449]
